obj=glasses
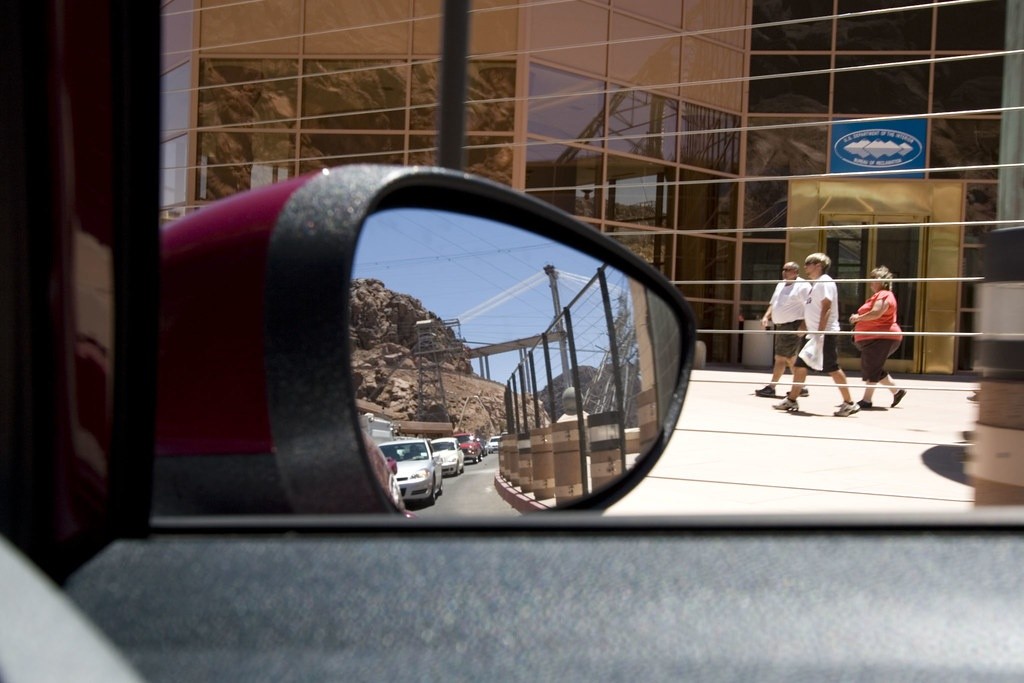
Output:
[804,262,814,266]
[782,268,794,272]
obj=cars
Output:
[432,437,466,477]
[487,435,502,454]
[378,438,444,508]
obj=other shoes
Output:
[755,386,776,397]
[857,400,872,409]
[966,395,979,402]
[890,389,907,408]
[787,390,809,397]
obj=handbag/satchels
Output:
[798,332,825,372]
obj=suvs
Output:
[453,433,483,465]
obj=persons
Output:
[849,266,907,410]
[771,253,861,416]
[755,262,809,397]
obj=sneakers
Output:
[834,401,861,416]
[772,398,798,412]
